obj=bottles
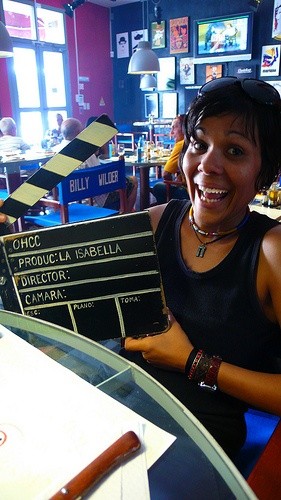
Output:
[149,114,154,123]
[47,128,63,147]
[138,135,155,161]
[108,141,115,155]
[259,182,281,207]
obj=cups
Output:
[164,149,170,154]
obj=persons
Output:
[0,77,281,452]
[0,116,138,212]
[152,114,191,203]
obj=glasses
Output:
[198,76,281,106]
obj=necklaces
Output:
[188,205,248,235]
[190,222,233,257]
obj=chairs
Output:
[0,132,188,233]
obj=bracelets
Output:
[185,348,222,392]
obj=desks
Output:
[123,160,168,211]
[0,149,57,194]
[0,309,260,500]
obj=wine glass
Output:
[118,144,125,160]
[156,141,164,161]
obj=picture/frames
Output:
[194,11,254,58]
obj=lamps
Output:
[127,0,160,89]
[0,19,13,57]
